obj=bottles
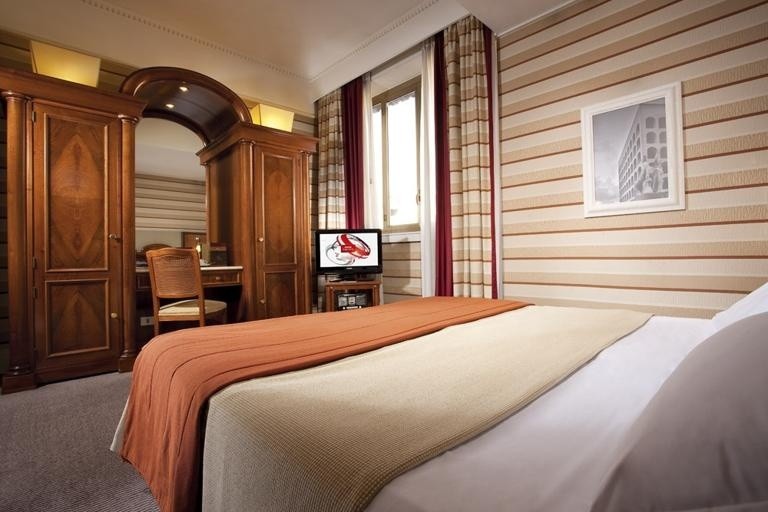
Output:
[194,237,201,259]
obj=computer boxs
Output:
[335,292,368,310]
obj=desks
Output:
[135,265,242,351]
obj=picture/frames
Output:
[578,81,686,218]
[180,230,209,262]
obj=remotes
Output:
[328,278,341,282]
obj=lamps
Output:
[246,103,295,134]
[27,40,99,89]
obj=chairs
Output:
[145,246,227,333]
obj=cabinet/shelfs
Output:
[247,140,306,323]
[30,98,123,383]
[324,280,381,312]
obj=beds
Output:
[110,282,768,511]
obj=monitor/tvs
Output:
[315,229,382,282]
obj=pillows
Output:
[584,285,767,511]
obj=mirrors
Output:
[129,115,209,262]
